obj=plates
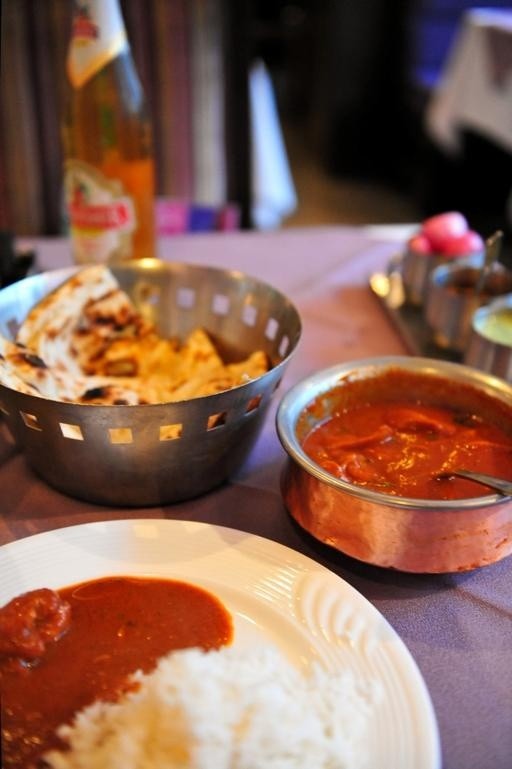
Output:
[0,517,441,769]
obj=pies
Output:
[0,264,268,444]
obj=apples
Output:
[422,211,470,250]
[410,235,433,256]
[444,230,483,258]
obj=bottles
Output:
[57,0,158,264]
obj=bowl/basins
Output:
[422,257,512,384]
[275,354,512,574]
[0,258,302,509]
